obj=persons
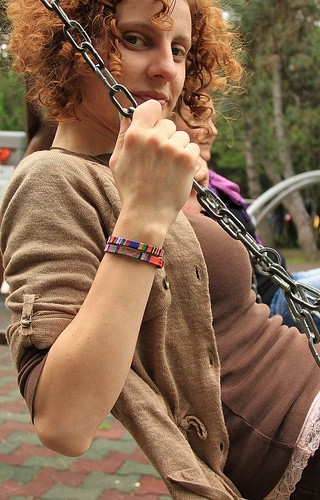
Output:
[0,1,320,500]
[172,90,320,344]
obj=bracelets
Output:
[104,244,165,268]
[107,234,165,258]
